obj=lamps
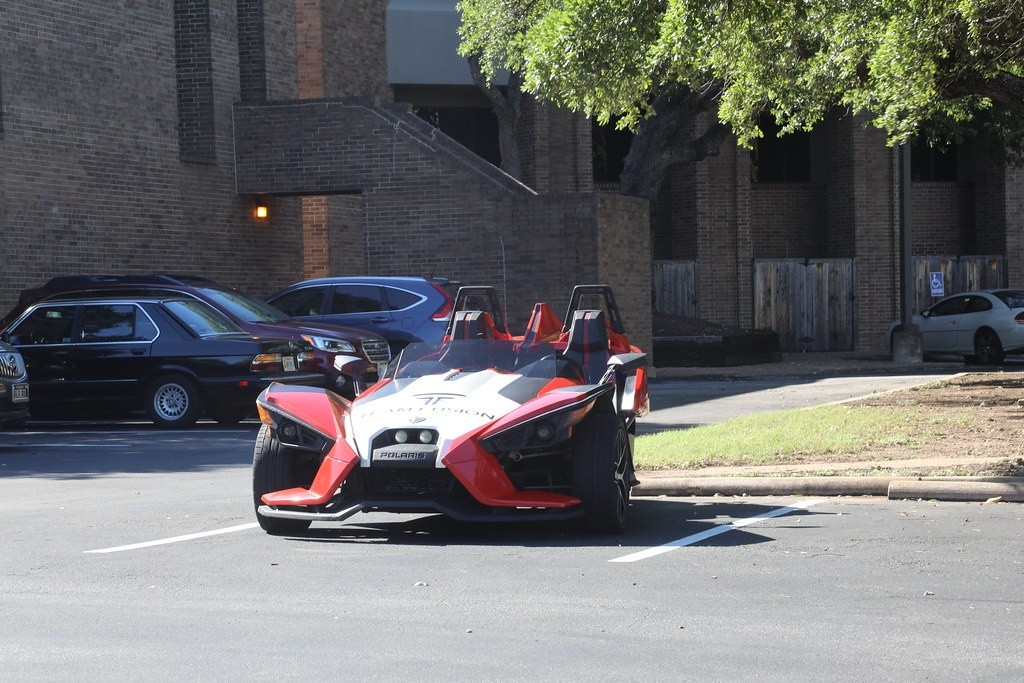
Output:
[256,202,267,219]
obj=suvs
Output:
[0,271,391,420]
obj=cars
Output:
[0,294,327,428]
[261,276,483,365]
[252,285,651,533]
[0,342,32,430]
[887,288,1024,365]
